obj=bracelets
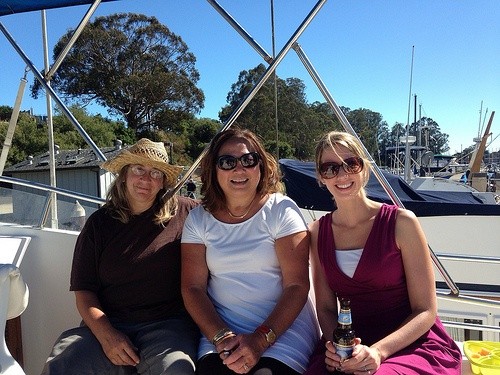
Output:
[212,328,237,346]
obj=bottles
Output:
[331,304,355,375]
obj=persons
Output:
[308,131,463,375]
[41,137,202,375]
[179,130,321,375]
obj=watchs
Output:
[255,325,276,346]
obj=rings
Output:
[244,365,248,370]
[364,366,368,371]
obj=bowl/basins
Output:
[463,340,500,375]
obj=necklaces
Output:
[226,205,251,218]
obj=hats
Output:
[98,138,185,191]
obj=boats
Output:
[279,44,500,344]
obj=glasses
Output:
[126,164,164,179]
[317,157,364,180]
[214,152,261,171]
